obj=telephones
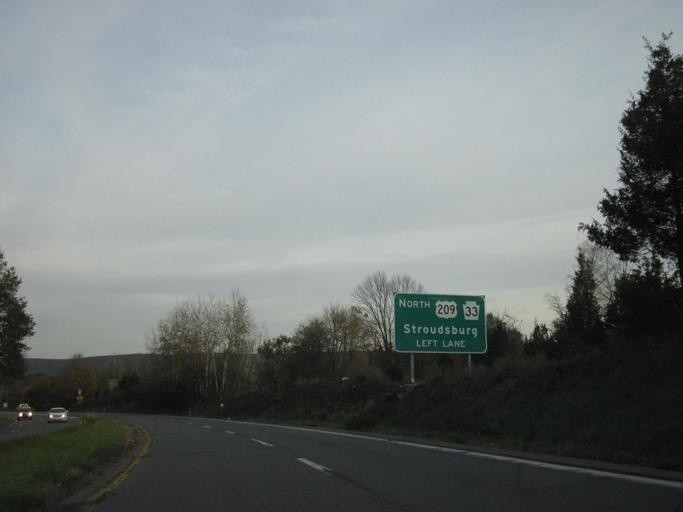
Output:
[391,293,488,354]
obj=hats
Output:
[16,403,33,420]
[48,406,69,423]
